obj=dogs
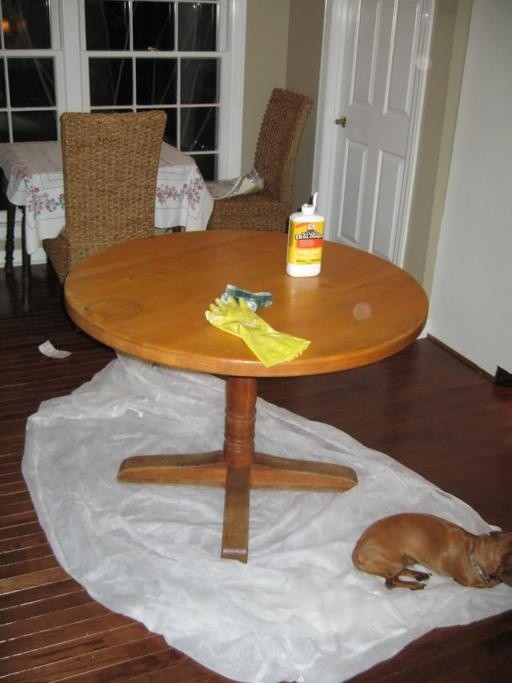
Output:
[352,512,512,591]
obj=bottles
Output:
[285,192,326,278]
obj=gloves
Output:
[204,297,312,368]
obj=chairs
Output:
[43,110,167,332]
[207,88,316,231]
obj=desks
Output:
[1,141,197,316]
[62,229,429,565]
